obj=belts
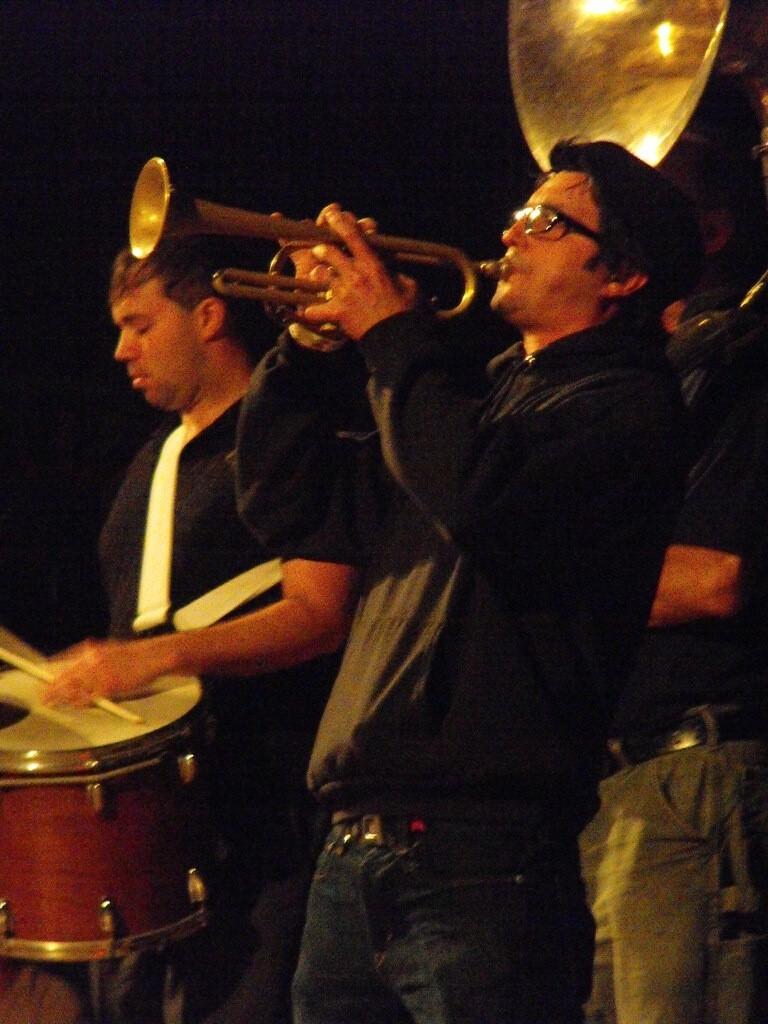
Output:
[598,709,768,772]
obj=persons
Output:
[233,140,699,1023]
[570,84,768,1024]
[59,226,382,1024]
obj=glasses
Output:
[508,202,598,242]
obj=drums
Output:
[0,642,209,963]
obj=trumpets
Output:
[130,159,513,337]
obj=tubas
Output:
[509,2,768,409]
